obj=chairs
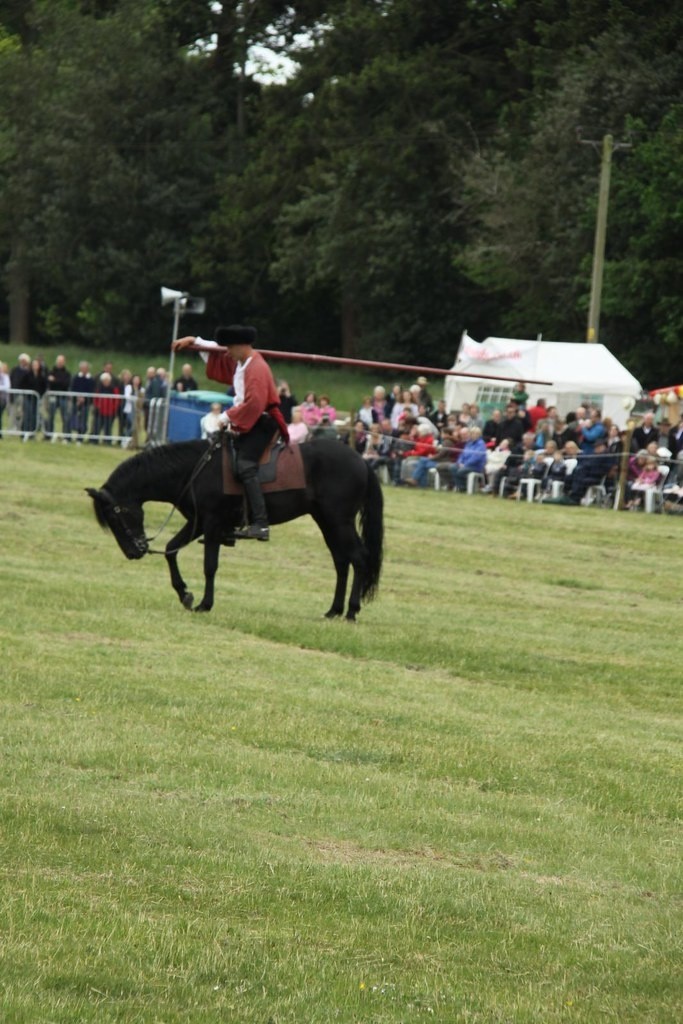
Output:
[326,407,678,512]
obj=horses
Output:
[82,436,384,625]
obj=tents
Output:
[649,386,683,426]
[444,330,644,432]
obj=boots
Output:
[198,538,235,546]
[234,477,269,541]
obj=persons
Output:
[0,355,196,450]
[171,324,290,541]
[200,376,683,510]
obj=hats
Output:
[215,324,257,345]
[416,376,428,384]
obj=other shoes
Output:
[535,493,551,500]
[480,485,496,492]
[508,493,521,499]
[407,478,418,486]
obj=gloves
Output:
[216,411,230,427]
[172,336,194,353]
